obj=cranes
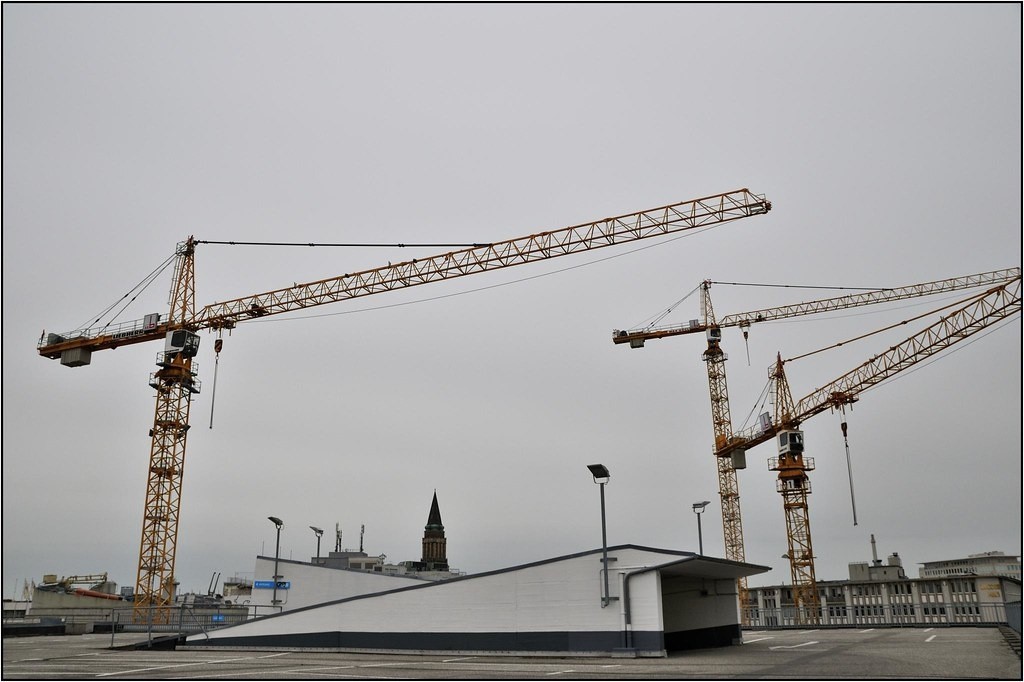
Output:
[36,189,772,626]
[712,278,1022,625]
[611,268,1021,626]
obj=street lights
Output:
[309,526,323,566]
[692,501,711,555]
[268,517,285,607]
[587,464,611,608]
